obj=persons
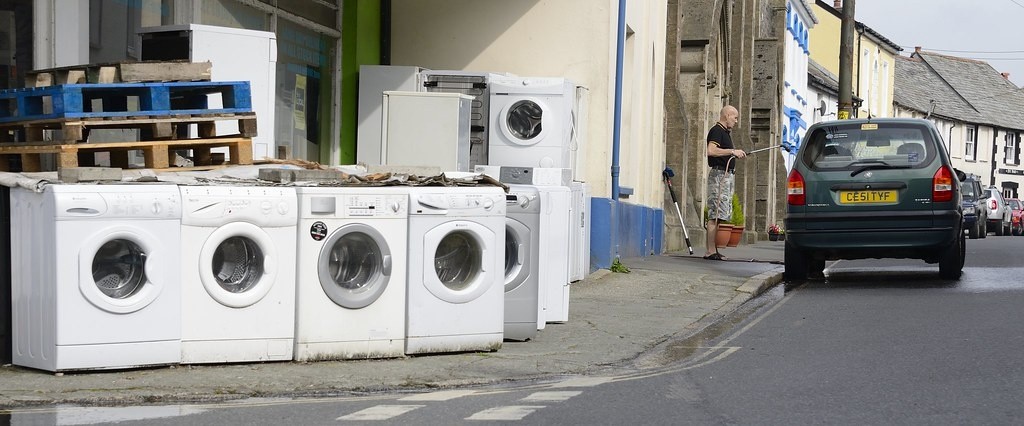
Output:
[703,104,747,261]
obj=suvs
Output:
[961,175,986,241]
[984,186,1013,235]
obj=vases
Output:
[778,234,785,241]
[769,234,779,241]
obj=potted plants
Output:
[704,206,734,249]
[728,192,745,247]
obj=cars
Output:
[1005,198,1024,235]
[783,116,967,282]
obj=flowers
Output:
[767,224,785,234]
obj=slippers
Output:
[703,252,727,261]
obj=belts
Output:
[712,165,736,174]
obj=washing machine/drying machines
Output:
[11,22,581,374]
